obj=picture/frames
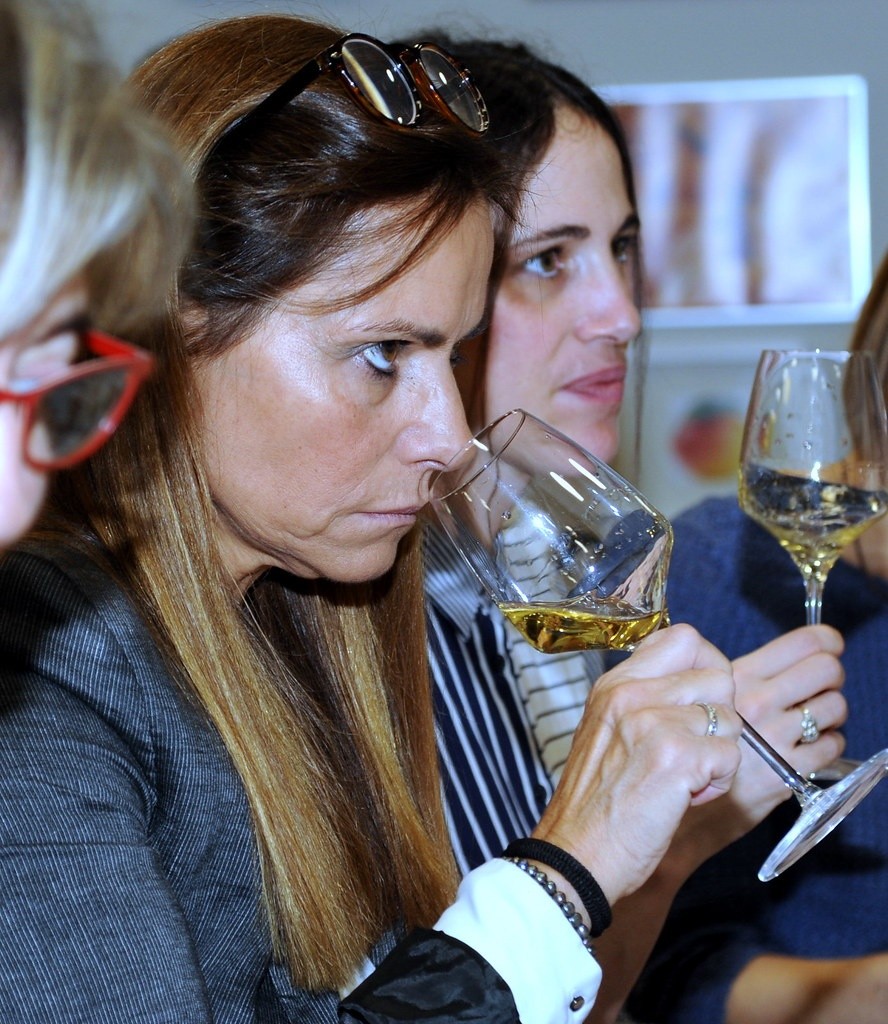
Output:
[583,75,873,330]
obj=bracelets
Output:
[503,838,612,937]
[505,857,595,957]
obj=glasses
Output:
[208,32,492,147]
[0,329,158,471]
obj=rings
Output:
[800,705,818,743]
[698,703,719,735]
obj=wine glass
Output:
[426,408,888,881]
[738,349,888,779]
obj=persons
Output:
[0,0,888,1023]
